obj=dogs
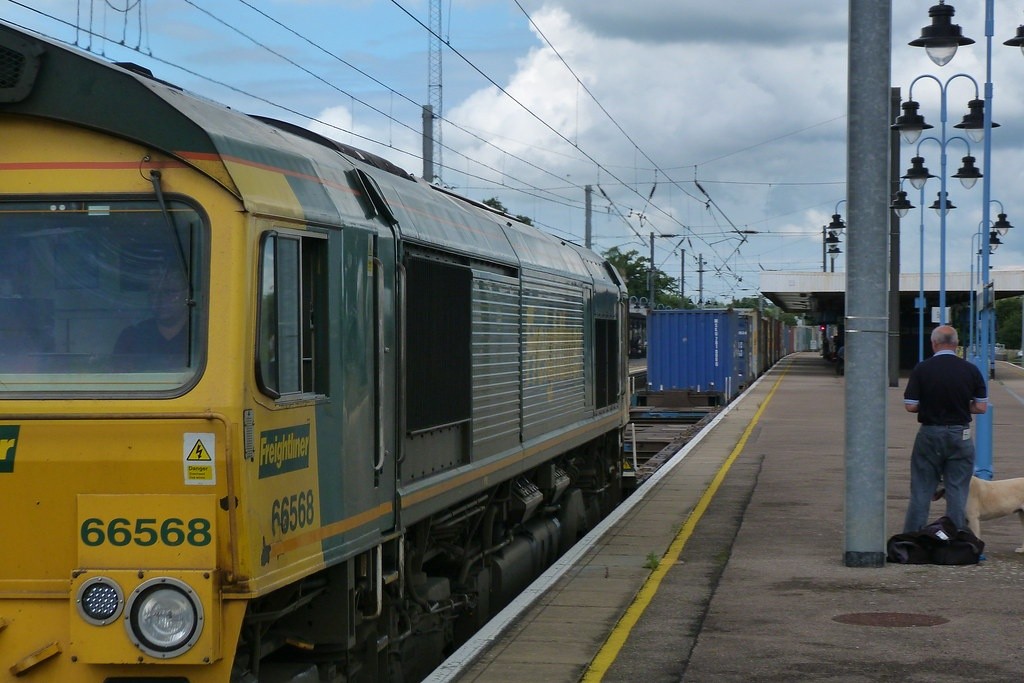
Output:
[933,474,1024,554]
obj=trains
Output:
[1,23,632,682]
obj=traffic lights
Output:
[819,325,826,332]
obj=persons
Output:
[108,268,190,369]
[822,334,845,375]
[903,325,989,533]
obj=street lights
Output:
[824,198,846,261]
[890,0,1024,480]
